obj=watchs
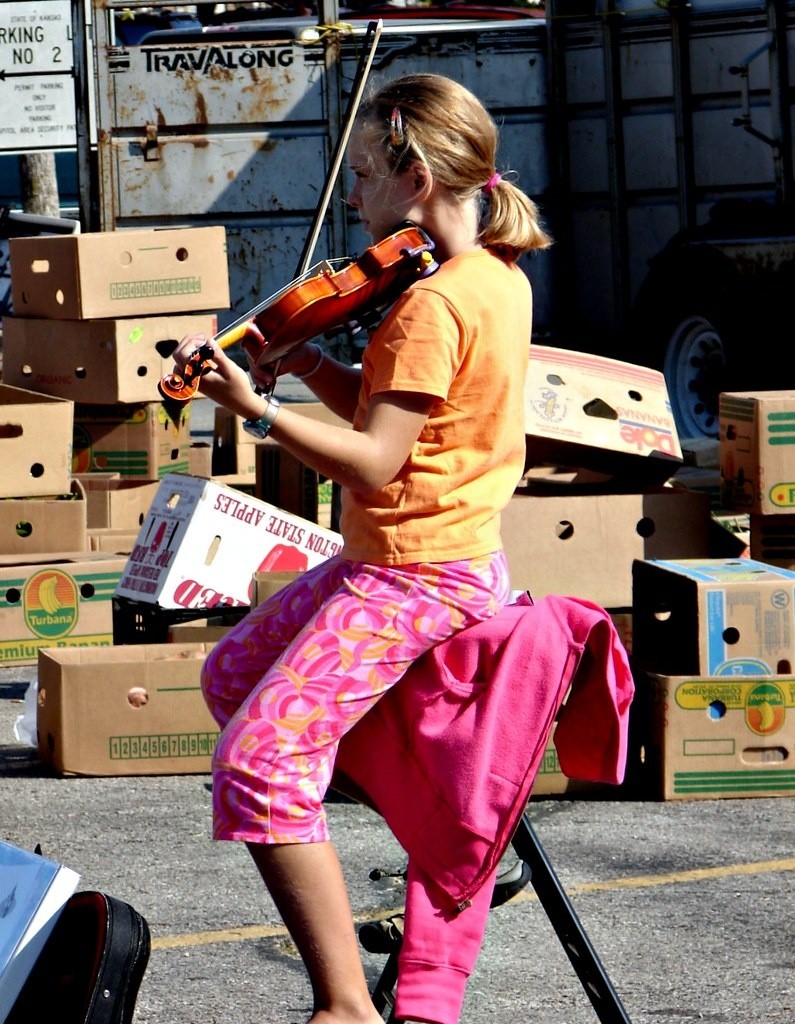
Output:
[243,394,280,439]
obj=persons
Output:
[173,75,554,1024]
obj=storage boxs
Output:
[1,840,82,1024]
[0,213,795,803]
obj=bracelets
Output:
[292,344,323,379]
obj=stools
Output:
[322,588,637,1024]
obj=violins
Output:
[158,227,434,406]
